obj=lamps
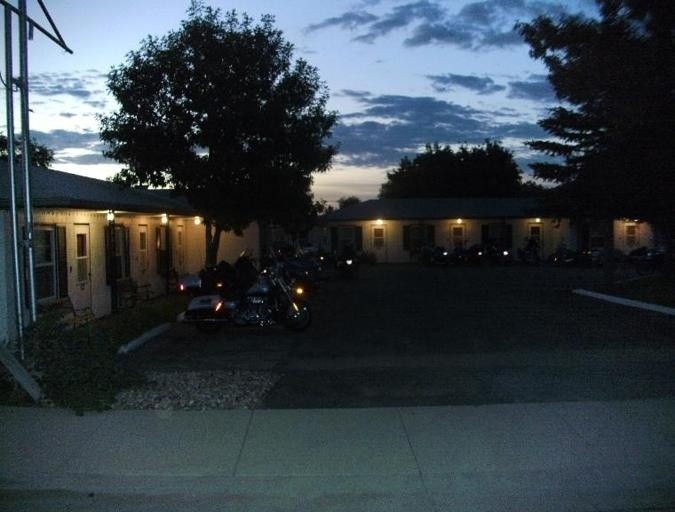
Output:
[107,208,115,221]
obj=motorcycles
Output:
[430,237,508,267]
[628,234,674,266]
[547,237,604,267]
[176,239,358,336]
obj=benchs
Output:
[110,276,155,313]
[34,296,95,339]
